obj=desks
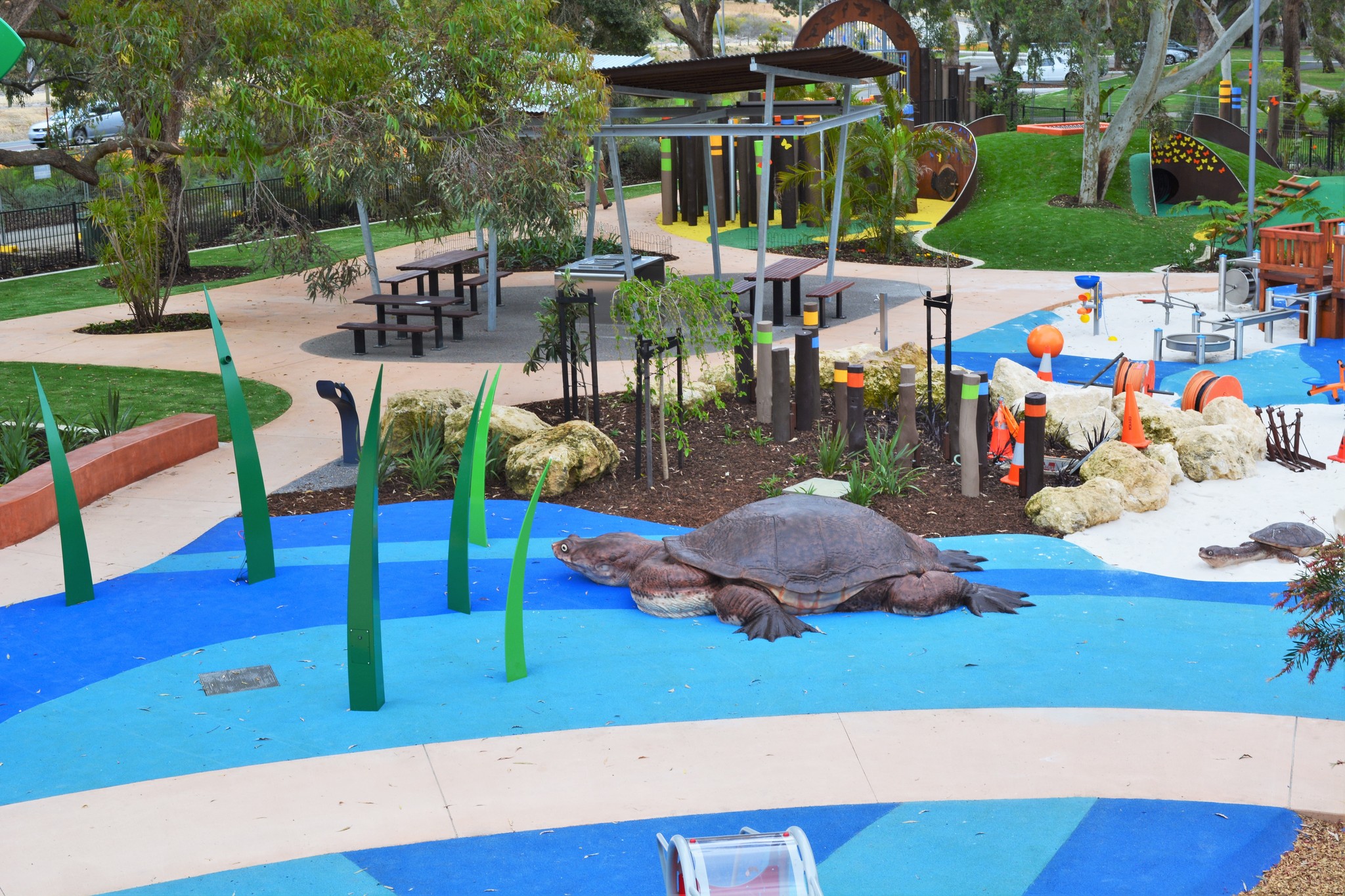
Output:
[744,258,827,327]
[554,255,665,323]
[353,294,465,351]
[396,249,489,308]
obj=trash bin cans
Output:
[77,206,142,259]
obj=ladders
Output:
[1206,176,1321,245]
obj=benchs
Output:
[806,280,855,328]
[337,270,514,358]
[722,277,768,317]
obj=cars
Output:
[1010,41,1109,86]
[28,99,126,149]
[1113,38,1198,65]
